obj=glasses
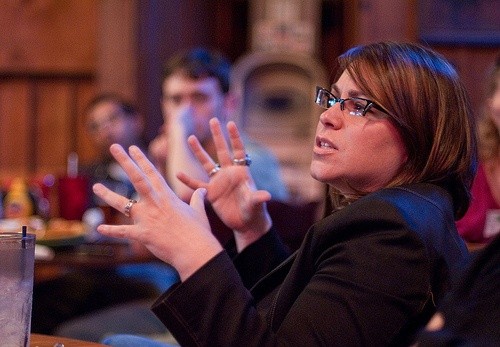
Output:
[314,85,391,117]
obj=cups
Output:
[0,233,36,347]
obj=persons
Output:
[53,45,500,347]
[92,39,479,347]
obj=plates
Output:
[38,235,86,247]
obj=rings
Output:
[123,199,137,218]
[207,163,221,177]
[232,153,253,167]
